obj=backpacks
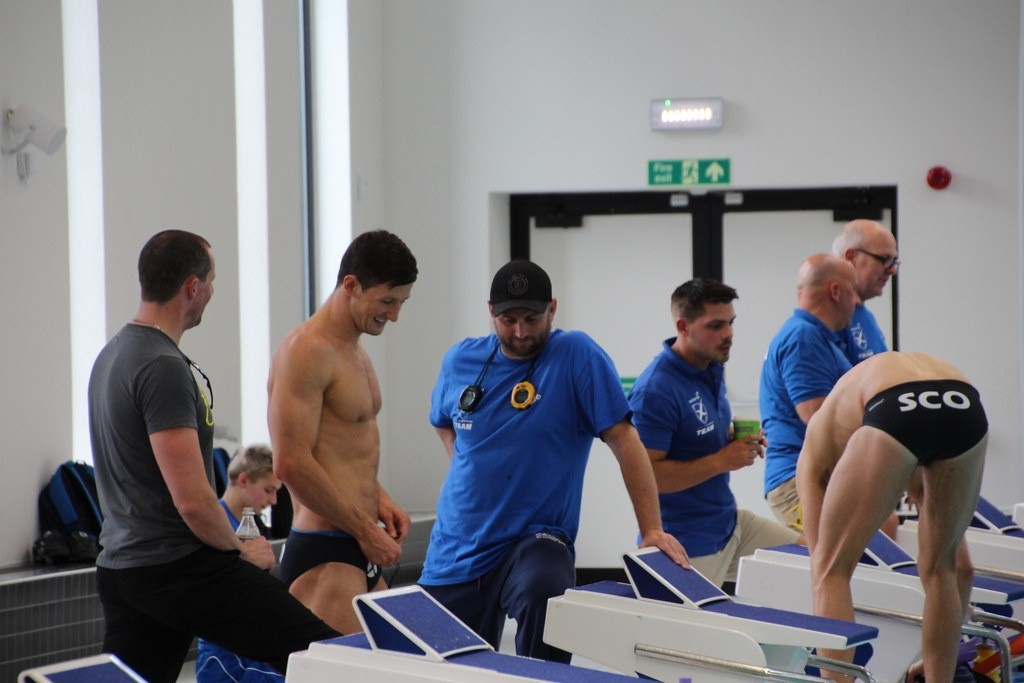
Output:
[33,460,103,567]
[213,446,229,498]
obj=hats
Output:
[490,257,553,317]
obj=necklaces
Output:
[132,319,164,332]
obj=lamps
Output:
[1,101,66,186]
[650,98,722,129]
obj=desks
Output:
[0,511,438,586]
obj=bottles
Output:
[236,507,260,540]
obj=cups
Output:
[732,415,760,440]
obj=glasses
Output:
[188,360,214,409]
[855,249,900,269]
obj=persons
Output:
[832,219,898,366]
[217,444,282,531]
[623,277,805,589]
[414,259,691,663]
[795,350,989,683]
[266,229,412,635]
[759,252,899,546]
[87,228,343,683]
